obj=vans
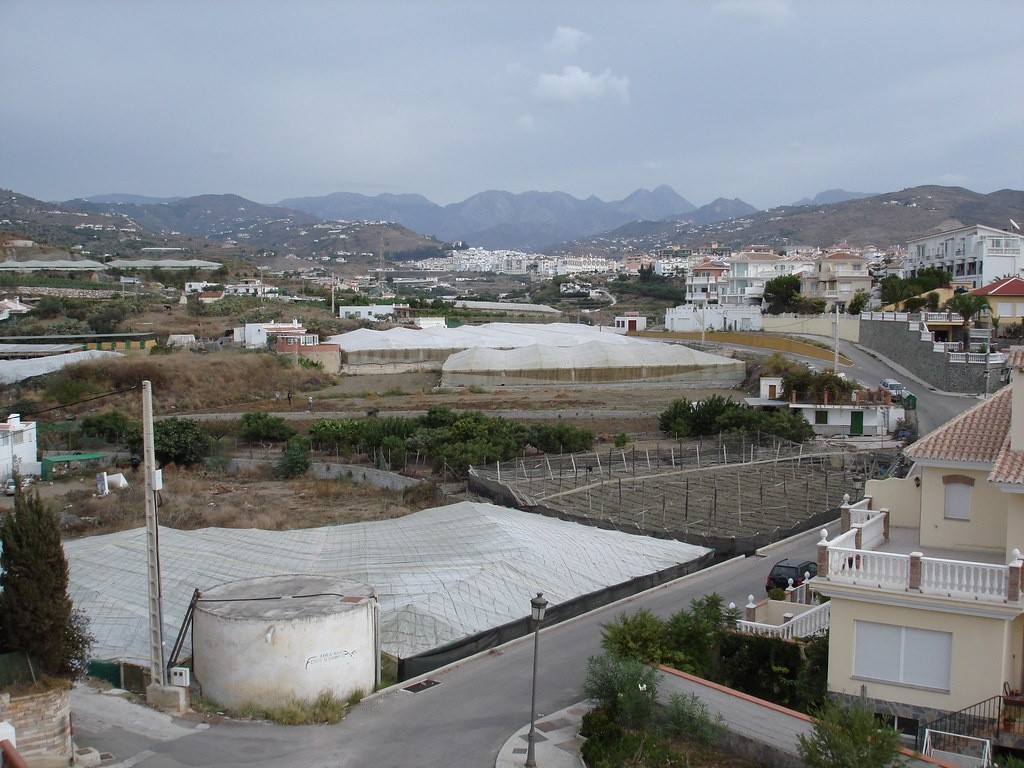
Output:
[879,378,907,402]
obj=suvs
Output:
[3,478,16,496]
[765,558,818,593]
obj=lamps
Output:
[914,476,921,488]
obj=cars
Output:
[22,477,36,488]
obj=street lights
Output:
[524,591,549,768]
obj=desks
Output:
[1003,692,1024,733]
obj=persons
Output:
[308,395,312,410]
[274,390,280,402]
[288,390,292,404]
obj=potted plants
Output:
[1002,716,1017,732]
